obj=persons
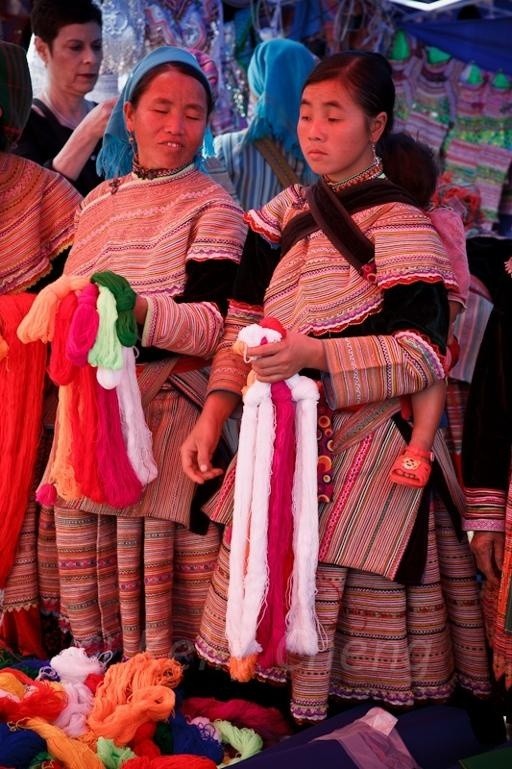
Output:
[464,222,511,725]
[16,0,122,192]
[213,39,327,215]
[0,48,84,658]
[370,129,470,486]
[181,50,490,722]
[3,44,252,667]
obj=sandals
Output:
[389,445,435,487]
